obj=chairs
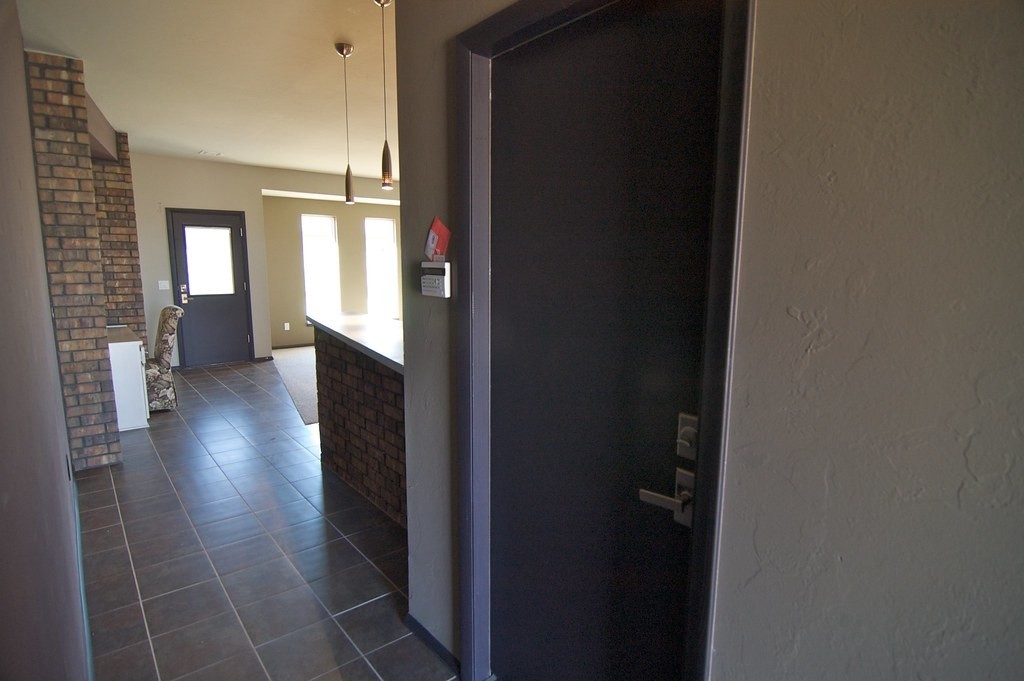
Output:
[145,304,185,412]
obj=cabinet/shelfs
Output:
[108,324,150,433]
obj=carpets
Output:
[272,345,318,426]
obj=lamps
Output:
[336,43,356,203]
[374,0,394,191]
